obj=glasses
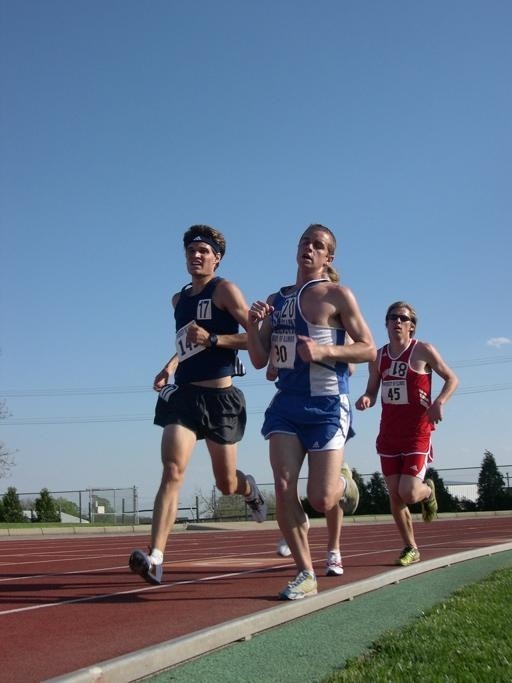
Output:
[388,313,412,322]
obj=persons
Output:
[356,300,461,568]
[244,220,378,602]
[127,223,267,586]
[265,266,356,579]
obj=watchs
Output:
[208,331,220,348]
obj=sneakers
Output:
[129,548,163,585]
[339,464,360,516]
[281,568,319,601]
[241,474,267,523]
[420,479,438,523]
[325,548,343,575]
[395,545,421,566]
[278,512,310,557]
